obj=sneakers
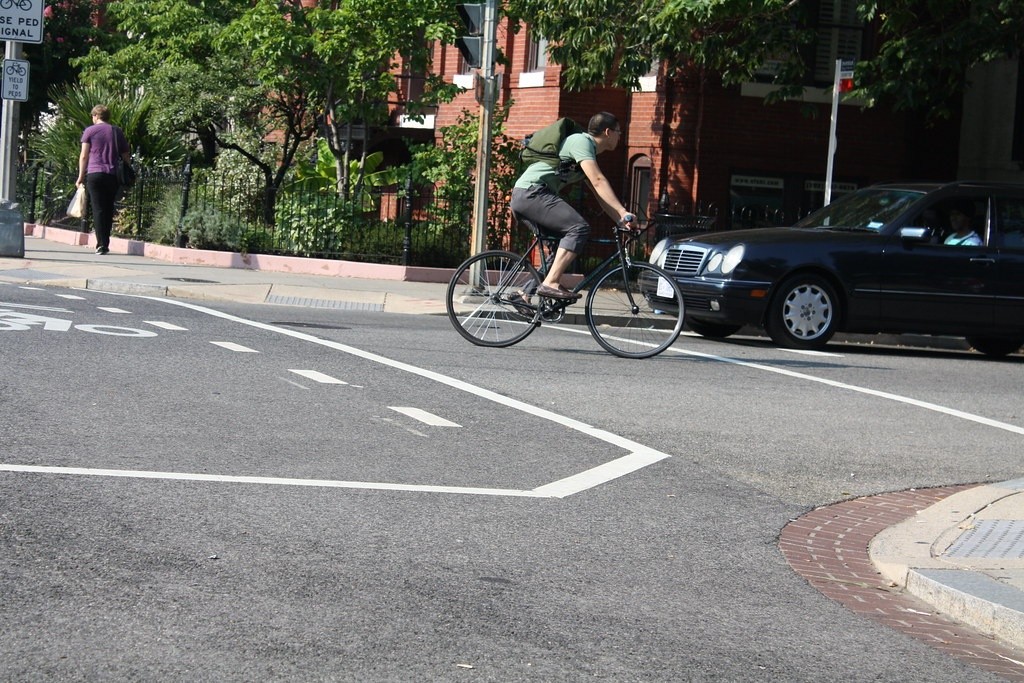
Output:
[95,245,109,254]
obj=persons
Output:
[76,106,130,255]
[944,202,983,245]
[511,113,638,317]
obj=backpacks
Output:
[518,117,586,171]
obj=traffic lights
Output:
[453,2,484,69]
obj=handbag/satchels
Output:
[112,126,135,188]
[66,182,85,218]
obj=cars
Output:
[638,164,1024,359]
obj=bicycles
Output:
[446,208,684,359]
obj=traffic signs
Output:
[840,60,855,81]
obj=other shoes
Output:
[511,292,541,326]
[538,284,582,298]
